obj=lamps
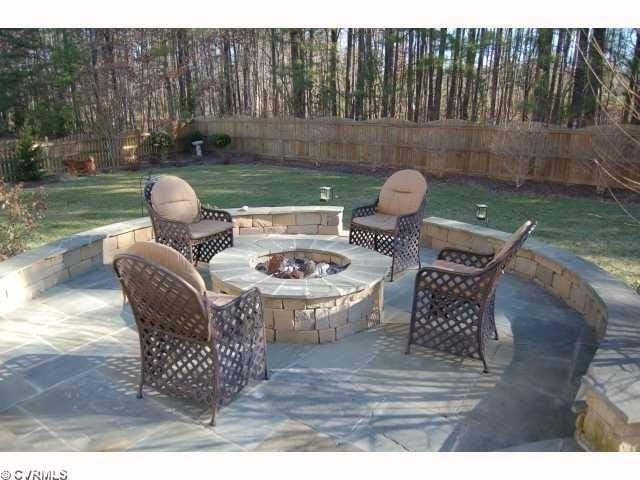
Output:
[320,186,334,203]
[474,204,489,224]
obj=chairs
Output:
[144,173,235,265]
[404,215,537,374]
[113,241,272,428]
[348,168,424,279]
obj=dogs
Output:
[61,155,97,177]
